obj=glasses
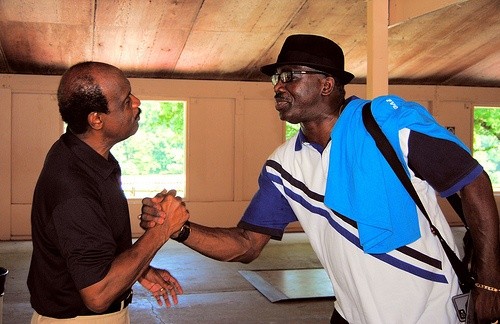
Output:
[271,70,330,86]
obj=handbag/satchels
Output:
[362,101,481,299]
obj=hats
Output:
[260,34,355,85]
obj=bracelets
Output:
[474,283,500,293]
[171,221,191,242]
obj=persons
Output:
[138,33,500,324]
[26,61,183,324]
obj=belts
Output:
[75,290,133,316]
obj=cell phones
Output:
[452,292,469,322]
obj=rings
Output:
[161,288,164,291]
[138,215,142,219]
